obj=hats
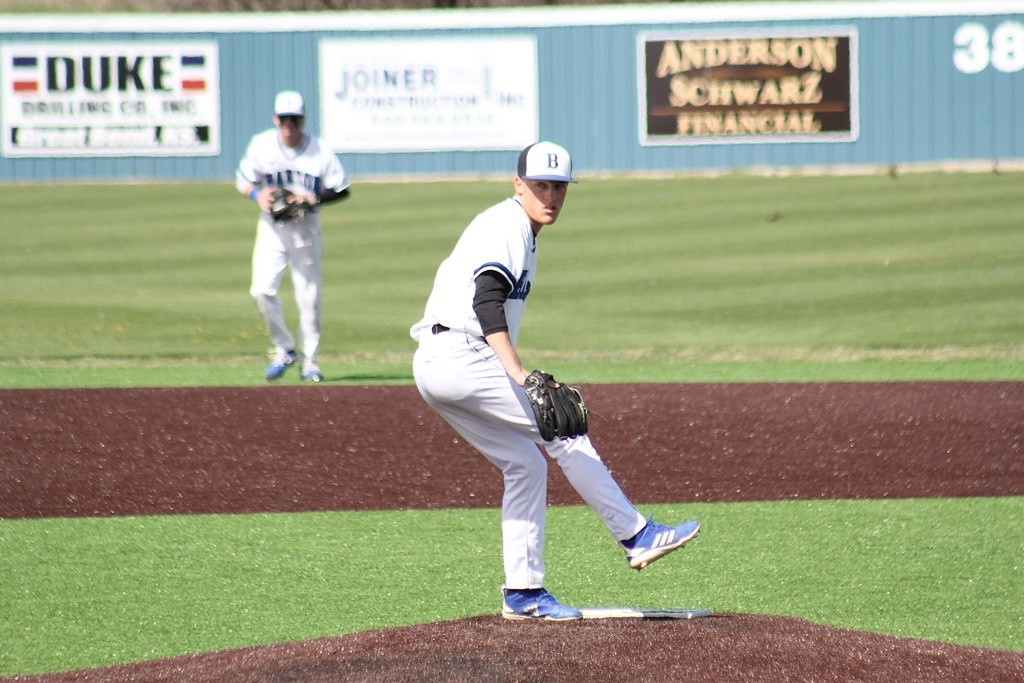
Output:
[273,90,304,116]
[517,142,578,183]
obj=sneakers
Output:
[264,350,298,381]
[500,585,583,621]
[618,513,701,570]
[298,351,323,383]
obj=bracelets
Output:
[249,187,260,202]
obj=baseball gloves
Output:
[267,188,315,221]
[523,368,591,441]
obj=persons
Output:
[235,90,351,386]
[409,140,702,623]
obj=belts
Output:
[431,324,450,334]
